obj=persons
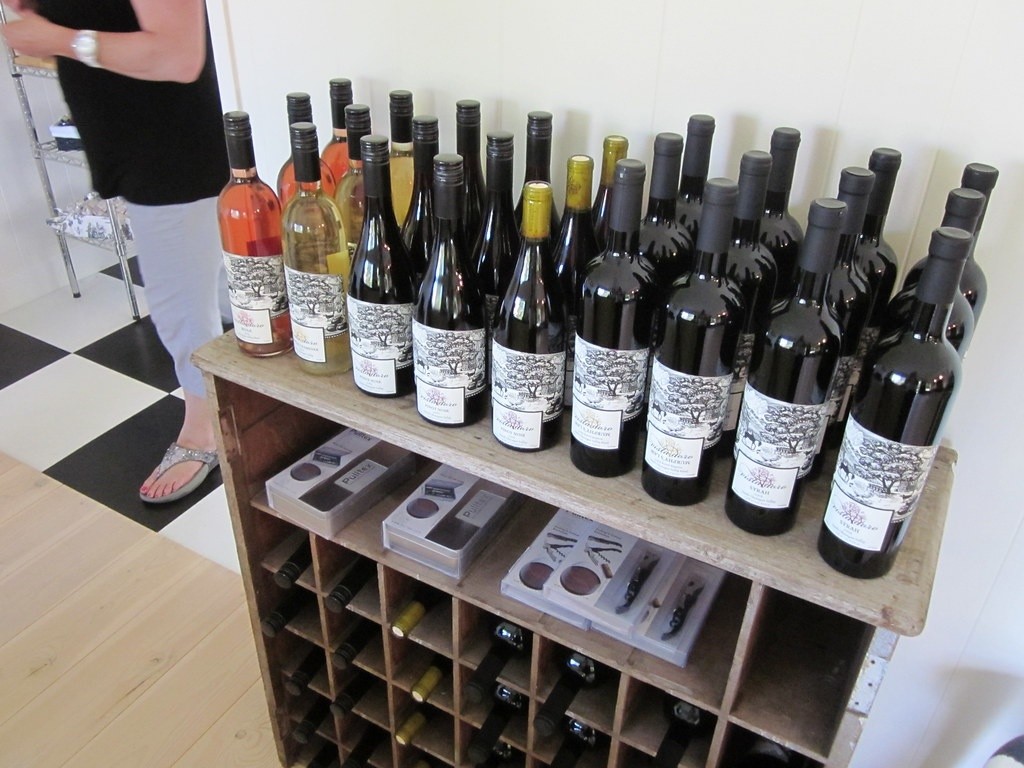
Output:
[0,1,234,506]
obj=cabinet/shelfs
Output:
[1,1,142,322]
[189,324,959,768]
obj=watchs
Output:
[70,28,103,69]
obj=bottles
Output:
[257,533,793,768]
[211,74,1010,579]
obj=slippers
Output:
[139,441,219,502]
